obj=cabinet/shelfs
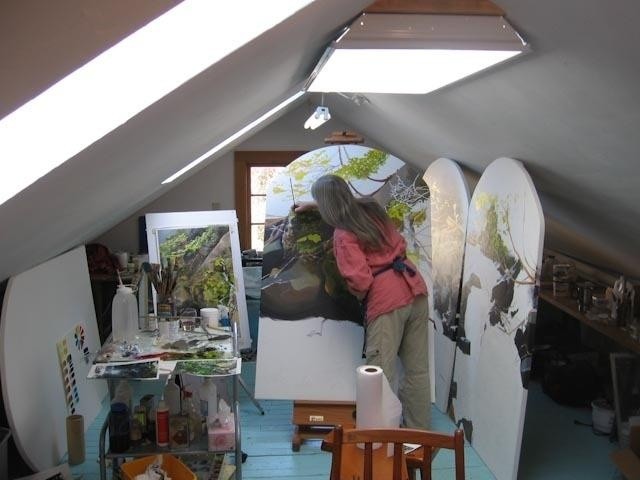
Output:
[85,317,245,479]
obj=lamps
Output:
[303,96,330,130]
[300,0,530,96]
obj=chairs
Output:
[332,424,465,480]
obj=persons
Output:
[290,173,433,435]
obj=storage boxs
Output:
[119,454,197,479]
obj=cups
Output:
[116,252,129,268]
[156,292,178,321]
[200,307,219,330]
[179,309,198,332]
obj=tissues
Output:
[206,398,239,451]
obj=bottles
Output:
[146,313,158,331]
[127,263,135,273]
[542,255,559,291]
[106,376,219,454]
[158,318,170,339]
[168,321,179,339]
[111,268,140,346]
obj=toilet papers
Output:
[357,365,402,449]
